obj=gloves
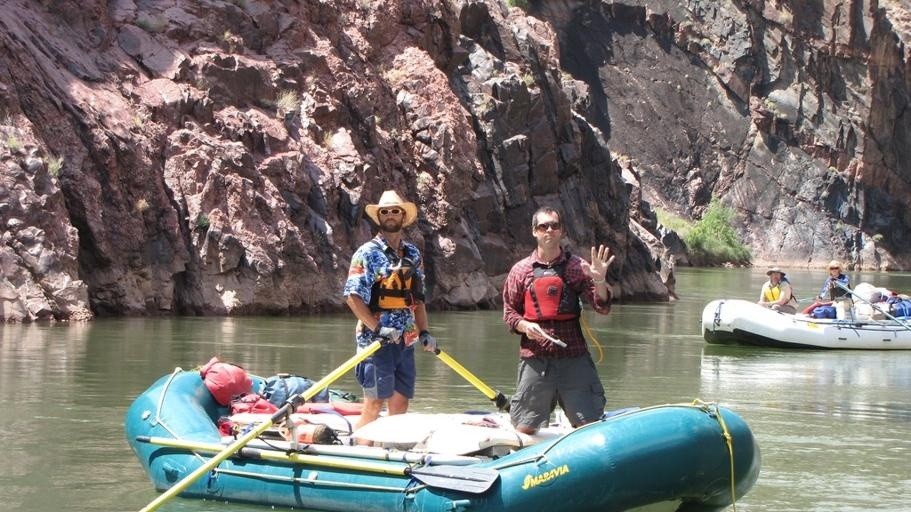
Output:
[418,330,436,352]
[375,323,400,344]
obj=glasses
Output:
[534,222,563,231]
[379,208,405,216]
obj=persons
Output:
[816,260,854,303]
[758,267,797,315]
[343,206,437,447]
[503,206,616,434]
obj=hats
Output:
[765,267,786,277]
[364,190,418,228]
[826,260,844,273]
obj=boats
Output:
[127,361,761,512]
[696,345,911,401]
[698,298,911,355]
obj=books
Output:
[533,325,567,348]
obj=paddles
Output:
[136,434,499,494]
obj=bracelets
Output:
[592,279,605,285]
